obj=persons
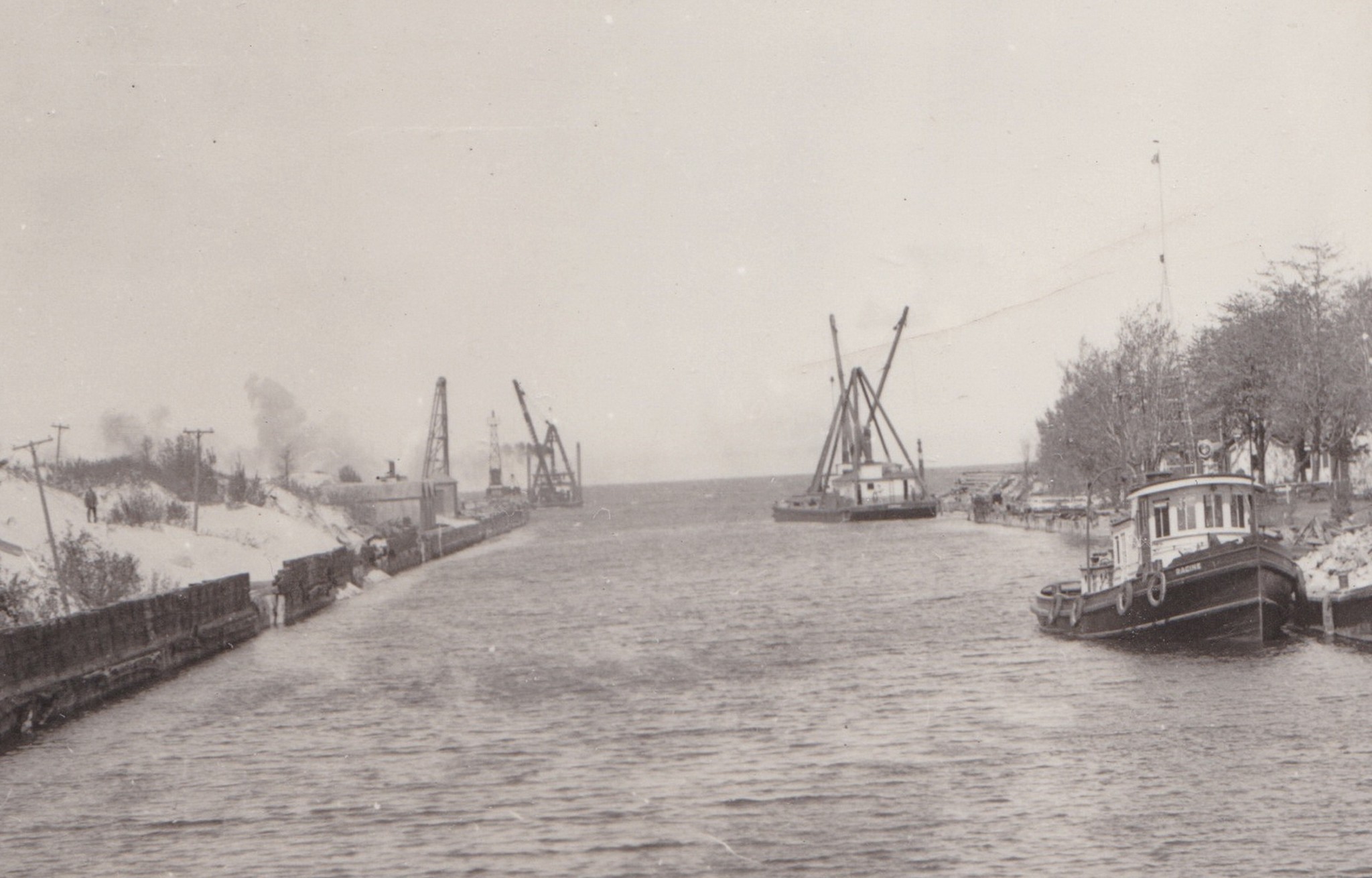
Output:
[973,490,1006,523]
[85,485,99,523]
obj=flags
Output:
[1151,152,1159,165]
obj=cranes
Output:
[422,377,449,477]
[485,410,510,495]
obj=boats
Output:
[1029,439,1301,645]
[772,367,938,522]
[525,424,584,507]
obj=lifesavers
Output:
[1147,572,1166,608]
[1197,442,1213,458]
[1048,596,1063,625]
[1070,597,1084,627]
[1115,581,1134,617]
